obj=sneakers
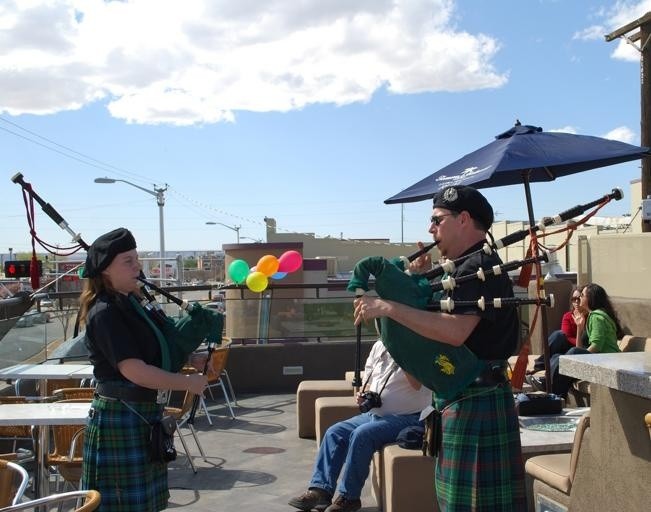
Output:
[288,488,333,512]
[324,495,361,512]
[526,374,547,391]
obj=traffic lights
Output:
[3,260,43,278]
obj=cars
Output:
[14,309,50,327]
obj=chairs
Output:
[525,414,591,512]
[508,334,651,407]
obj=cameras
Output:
[358,390,382,414]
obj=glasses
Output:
[570,297,580,303]
[431,213,450,225]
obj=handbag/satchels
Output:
[149,416,178,463]
[420,406,441,457]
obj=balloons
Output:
[228,249,303,292]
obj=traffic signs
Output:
[77,266,88,280]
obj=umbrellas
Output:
[384,117,651,394]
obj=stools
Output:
[298,365,439,511]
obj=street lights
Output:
[204,220,241,244]
[92,175,169,316]
[239,236,262,244]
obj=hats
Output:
[81,228,137,277]
[395,426,424,450]
[433,185,494,223]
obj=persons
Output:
[287,334,433,512]
[525,285,590,381]
[552,284,625,402]
[352,184,530,512]
[74,226,211,511]
